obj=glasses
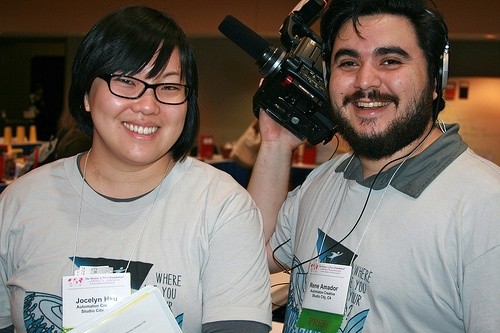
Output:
[96,72,195,106]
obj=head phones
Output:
[321,7,450,91]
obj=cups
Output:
[199,135,213,159]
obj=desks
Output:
[192,154,321,191]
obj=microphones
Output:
[219,15,273,61]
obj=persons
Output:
[247,0,500,332]
[0,5,272,333]
